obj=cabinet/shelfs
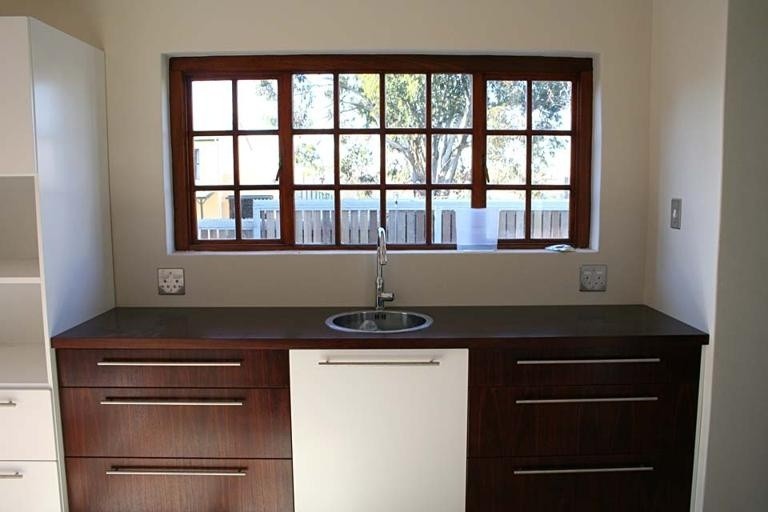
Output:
[1,15,117,511]
[54,347,701,511]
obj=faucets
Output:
[374,227,396,310]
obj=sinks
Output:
[325,310,435,334]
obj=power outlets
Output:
[156,267,186,296]
[579,264,608,291]
